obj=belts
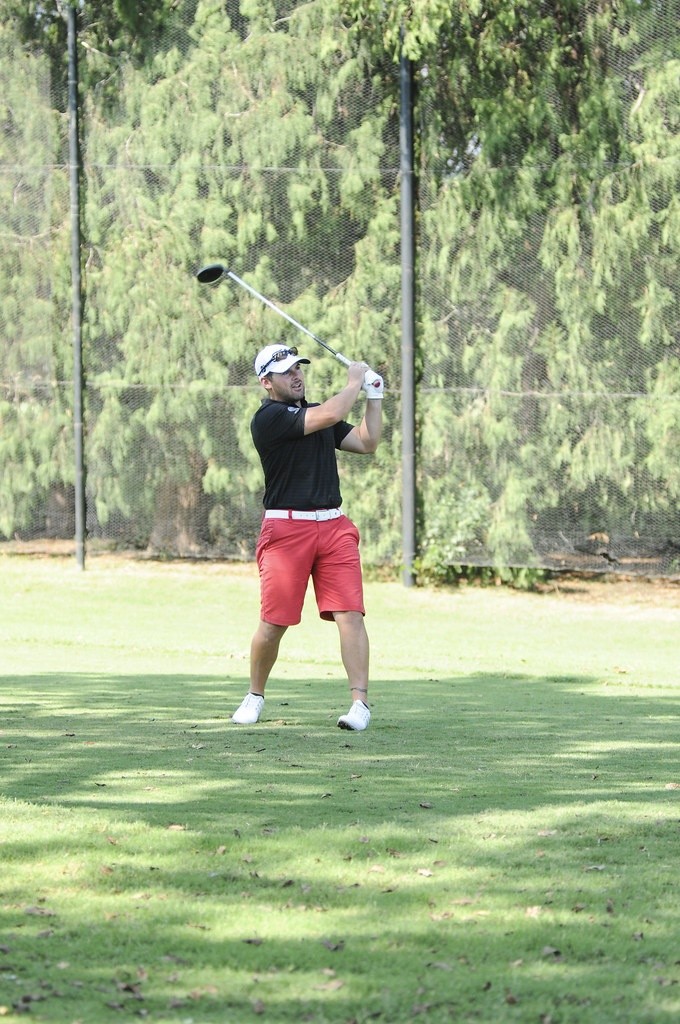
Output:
[264,507,344,521]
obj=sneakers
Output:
[232,692,265,724]
[336,698,371,731]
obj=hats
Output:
[254,343,311,380]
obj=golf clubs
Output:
[196,263,382,390]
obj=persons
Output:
[233,343,384,729]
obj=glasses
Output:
[258,346,298,376]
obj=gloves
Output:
[361,369,384,399]
